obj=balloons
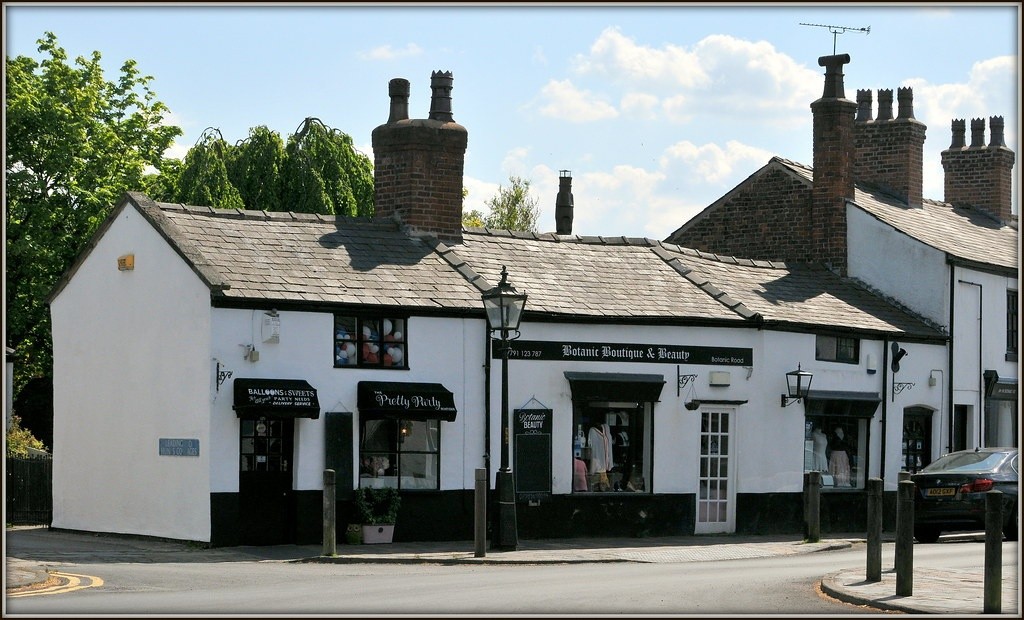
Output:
[334,319,404,367]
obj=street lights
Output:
[482,264,529,552]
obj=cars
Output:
[910,447,1018,542]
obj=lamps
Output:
[677,364,700,411]
[781,362,813,408]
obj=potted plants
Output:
[348,487,399,544]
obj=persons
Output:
[811,427,851,487]
[360,456,373,474]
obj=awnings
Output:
[233,378,322,420]
[357,382,457,422]
